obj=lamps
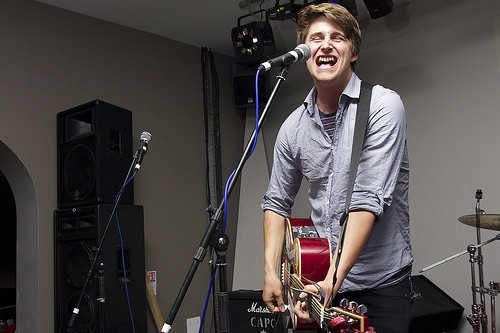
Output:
[314,0,394,19]
[231,0,307,65]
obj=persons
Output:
[261,3,415,333]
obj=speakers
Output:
[231,58,267,108]
[406,274,466,333]
[217,291,289,333]
[55,98,134,208]
[54,204,148,333]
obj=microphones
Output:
[258,43,312,72]
[136,131,153,171]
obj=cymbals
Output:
[458,214,500,231]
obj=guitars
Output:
[280,216,377,333]
